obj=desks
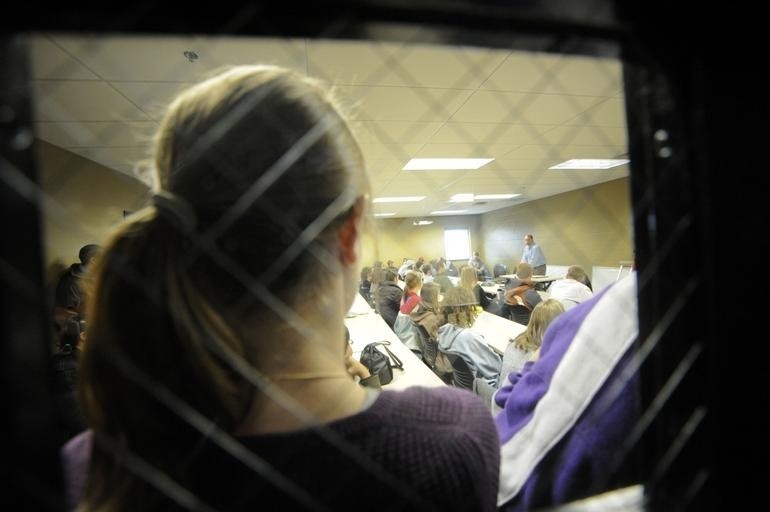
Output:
[343,269,560,413]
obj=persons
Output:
[491,268,643,512]
[50,244,103,370]
[59,65,499,511]
[345,235,596,392]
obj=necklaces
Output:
[245,370,348,380]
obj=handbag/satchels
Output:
[358,340,405,386]
